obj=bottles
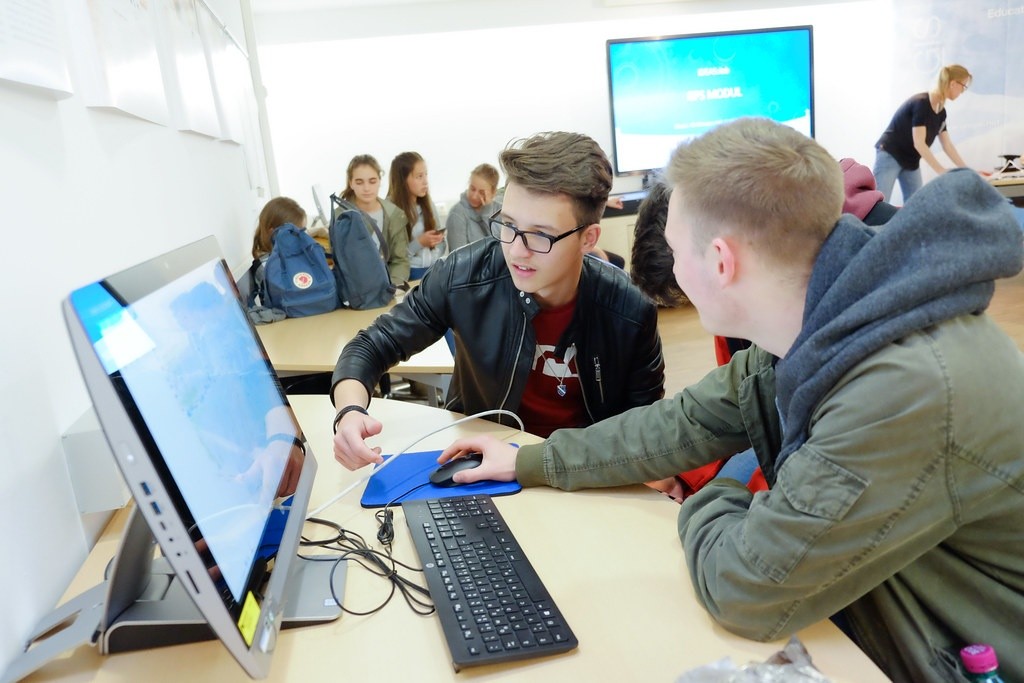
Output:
[960,644,1010,683]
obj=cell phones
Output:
[435,226,447,235]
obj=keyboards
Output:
[402,493,578,674]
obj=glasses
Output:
[956,81,968,91]
[488,208,588,254]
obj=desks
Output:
[14,394,893,683]
[253,287,455,409]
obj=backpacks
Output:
[261,224,343,318]
[329,192,397,310]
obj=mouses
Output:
[429,453,485,488]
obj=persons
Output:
[323,152,411,299]
[587,194,626,271]
[445,162,505,254]
[434,116,1024,682]
[236,196,318,326]
[329,129,669,473]
[627,156,894,508]
[872,63,974,207]
[384,151,450,283]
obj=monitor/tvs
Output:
[606,25,815,186]
[61,234,345,681]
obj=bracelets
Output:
[333,405,369,436]
